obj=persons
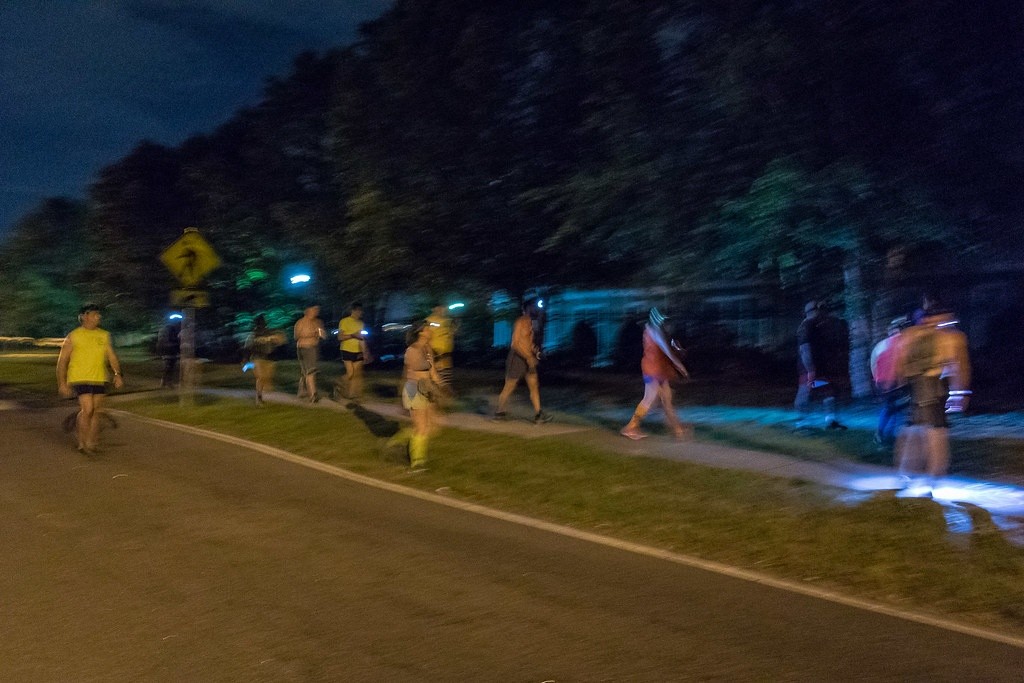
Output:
[294,302,326,403]
[425,297,456,410]
[619,284,692,441]
[156,319,179,390]
[866,297,972,494]
[334,302,370,401]
[792,297,853,436]
[56,302,125,452]
[492,287,556,424]
[376,319,445,484]
[244,312,284,408]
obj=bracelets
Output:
[113,370,121,376]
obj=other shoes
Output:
[348,394,364,404]
[407,463,432,474]
[297,391,311,398]
[620,426,649,441]
[492,410,512,419]
[255,399,267,409]
[676,429,693,442]
[534,410,554,423]
[377,447,394,462]
[78,445,87,453]
[310,393,322,403]
[332,379,343,402]
[795,420,812,429]
[824,414,847,430]
[86,442,102,453]
